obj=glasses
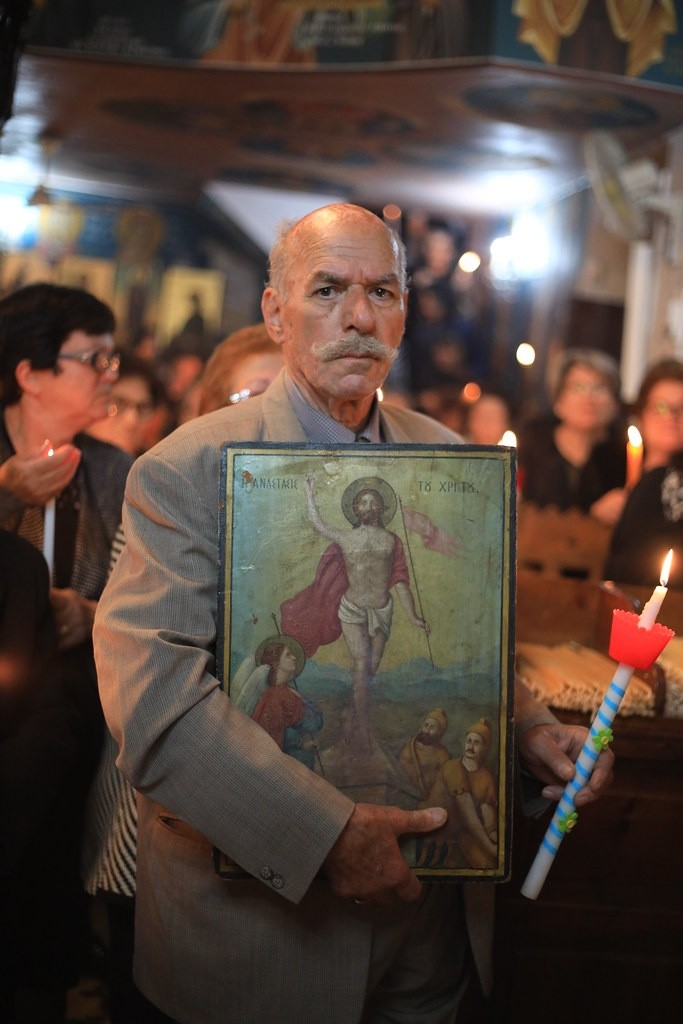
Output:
[107,396,155,417]
[56,351,121,374]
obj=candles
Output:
[521,583,668,900]
[42,497,56,589]
[627,442,644,488]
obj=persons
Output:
[0,273,682,1024]
[85,207,489,1024]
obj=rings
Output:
[352,896,373,905]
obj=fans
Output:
[584,128,683,265]
